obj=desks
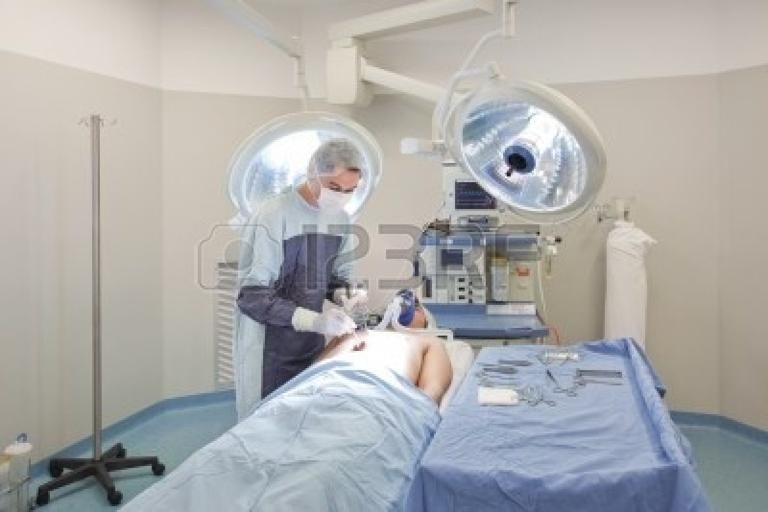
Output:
[426,340,715,512]
[423,303,550,354]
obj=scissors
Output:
[553,383,580,397]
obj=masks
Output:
[316,186,355,218]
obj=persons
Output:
[120,289,456,512]
[232,138,365,417]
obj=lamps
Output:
[226,0,607,225]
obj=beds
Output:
[120,332,476,512]
